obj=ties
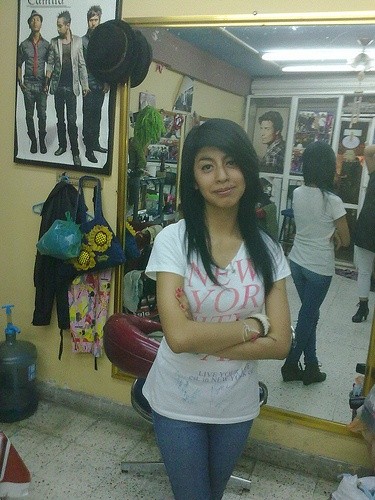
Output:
[32,40,40,80]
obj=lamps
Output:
[255,37,375,128]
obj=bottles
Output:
[0,328,38,422]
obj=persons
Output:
[16,10,49,154]
[81,5,108,163]
[352,144,375,323]
[258,111,286,174]
[258,178,273,198]
[141,118,293,500]
[43,10,89,166]
[297,112,327,132]
[280,142,349,386]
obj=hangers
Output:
[31,176,94,225]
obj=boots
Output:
[303,357,326,385]
[281,345,304,382]
[352,300,369,322]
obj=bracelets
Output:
[19,80,22,83]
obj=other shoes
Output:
[40,141,47,154]
[30,141,37,153]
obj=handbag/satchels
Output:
[35,212,83,260]
[62,175,127,279]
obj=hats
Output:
[87,19,137,83]
[28,9,44,22]
[130,30,152,88]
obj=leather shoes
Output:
[93,147,108,152]
[85,151,98,163]
[55,147,66,155]
[73,155,81,166]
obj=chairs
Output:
[102,311,269,492]
[279,186,301,243]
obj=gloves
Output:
[246,313,295,348]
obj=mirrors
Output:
[103,10,375,440]
